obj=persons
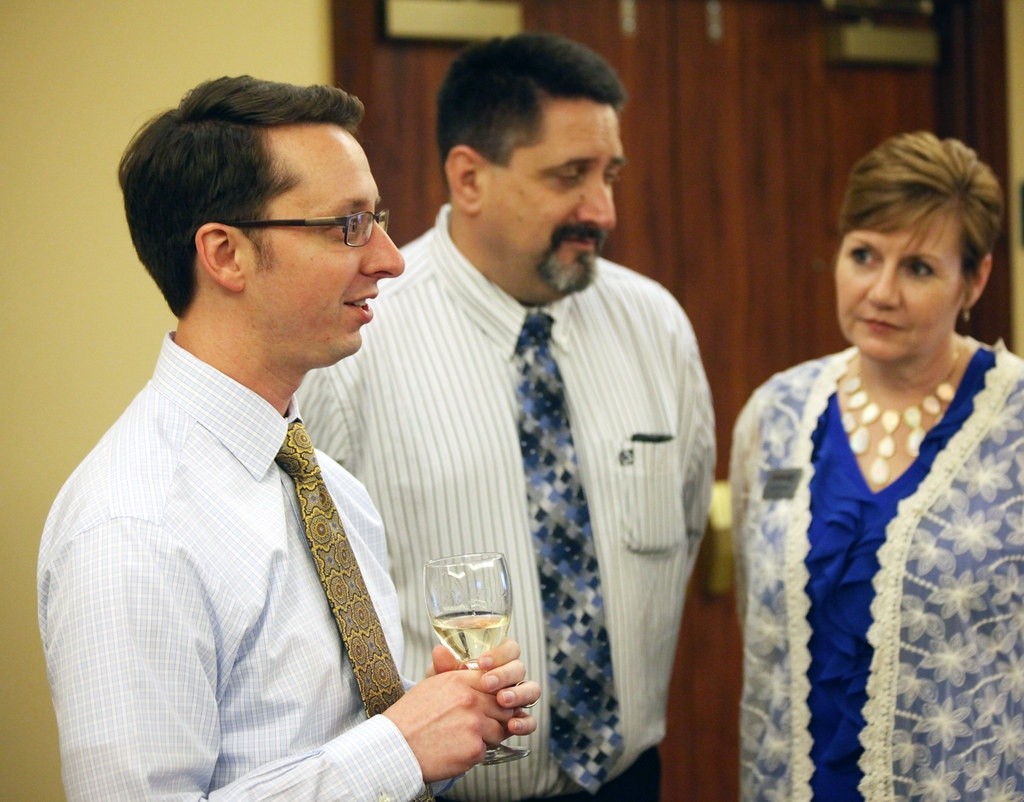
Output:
[296,36,716,802]
[729,131,1024,802]
[36,76,540,802]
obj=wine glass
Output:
[424,552,531,765]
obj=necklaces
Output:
[840,365,956,486]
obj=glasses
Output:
[220,208,390,247]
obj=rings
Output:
[516,680,538,708]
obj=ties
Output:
[512,313,625,795]
[273,421,441,802]
